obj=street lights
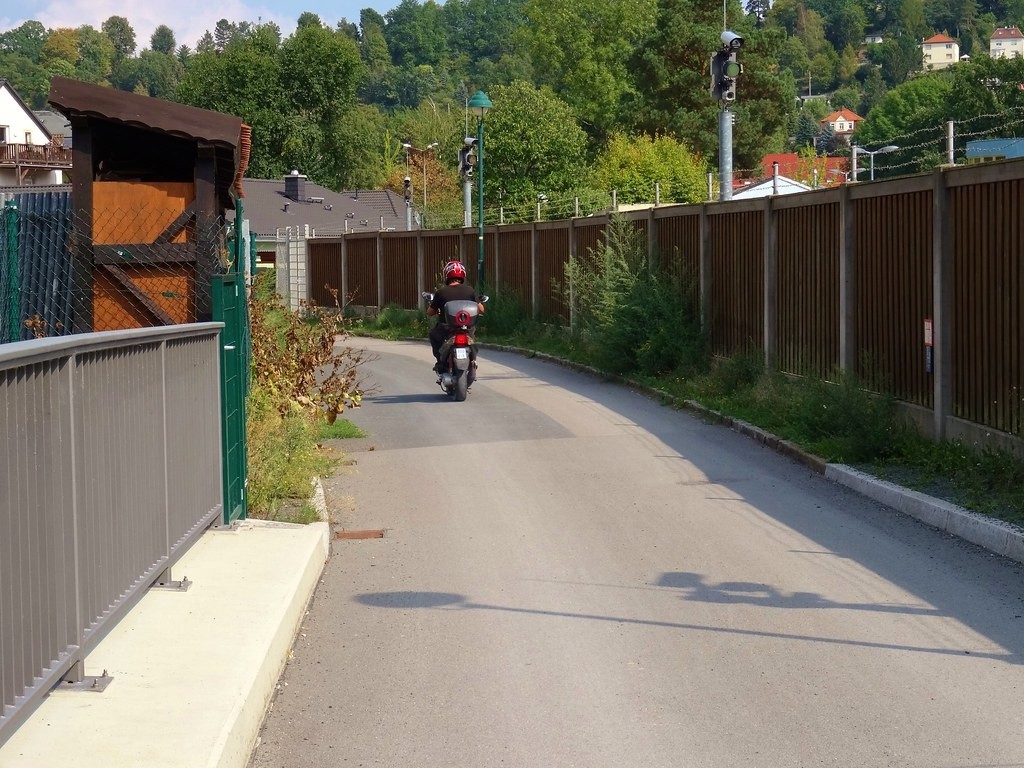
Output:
[466,89,495,302]
[828,168,865,186]
[404,143,438,208]
[857,145,899,180]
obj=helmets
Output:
[443,261,467,285]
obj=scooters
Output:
[421,291,490,401]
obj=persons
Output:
[427,261,485,370]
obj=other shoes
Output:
[433,362,448,373]
[471,360,478,369]
